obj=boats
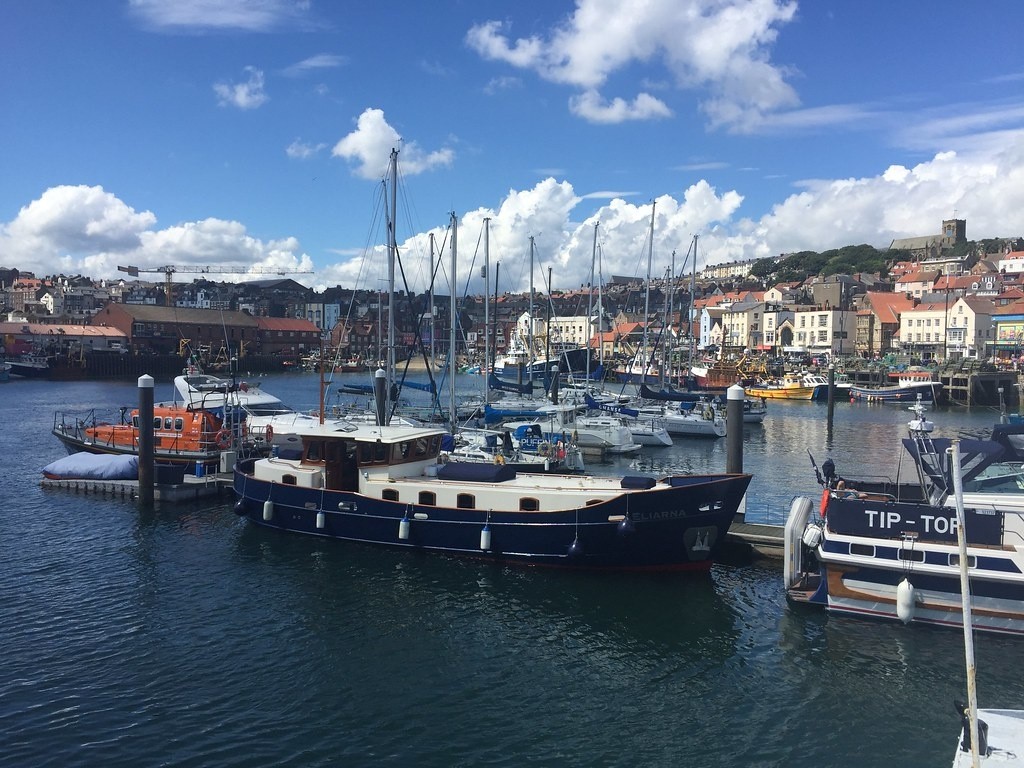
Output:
[784,392,1024,639]
[50,374,302,466]
[746,372,852,402]
[335,355,366,371]
[850,382,943,408]
[0,320,157,381]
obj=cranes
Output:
[117,265,315,306]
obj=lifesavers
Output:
[539,441,552,455]
[571,429,579,442]
[265,425,272,445]
[216,426,232,449]
[721,408,727,419]
[493,454,505,466]
[703,410,712,420]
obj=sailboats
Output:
[231,137,754,575]
[402,198,766,472]
